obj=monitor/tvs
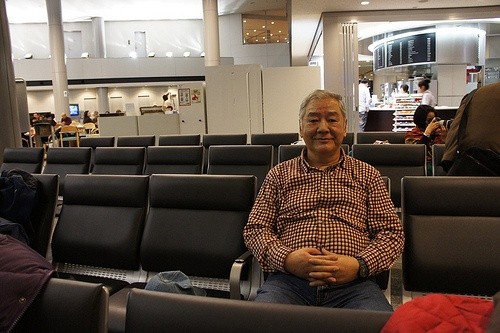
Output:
[69,104,79,116]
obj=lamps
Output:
[183,52,190,58]
[25,54,32,59]
[81,53,89,58]
[149,53,155,57]
[201,53,205,57]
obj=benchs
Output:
[0,134,500,333]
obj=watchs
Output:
[356,256,368,280]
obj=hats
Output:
[143,269,207,299]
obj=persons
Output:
[241,87,405,314]
[30,109,123,136]
[404,105,451,175]
[441,82,500,174]
[162,94,172,111]
[400,84,409,96]
[418,80,436,110]
[358,78,371,132]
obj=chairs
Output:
[34,121,99,147]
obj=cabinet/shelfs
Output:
[391,97,422,133]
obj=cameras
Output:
[435,117,453,128]
